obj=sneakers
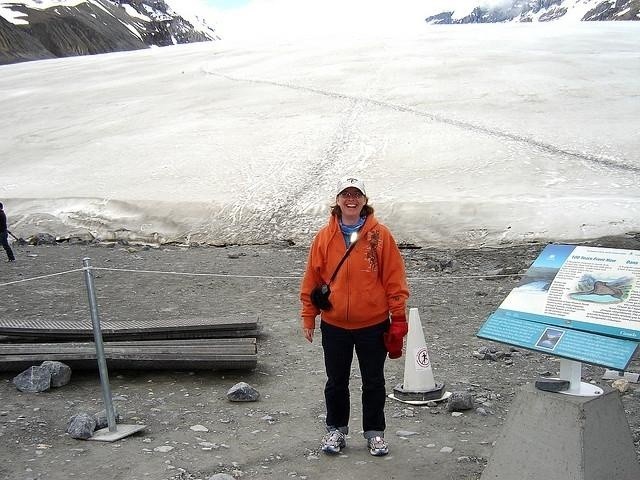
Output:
[320,428,348,455]
[366,437,391,457]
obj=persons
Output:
[300,174,412,455]
[0,202,15,262]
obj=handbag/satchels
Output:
[309,283,335,313]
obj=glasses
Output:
[338,190,363,199]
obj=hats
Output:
[335,175,369,200]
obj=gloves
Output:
[383,330,404,361]
[386,312,410,341]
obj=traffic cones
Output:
[392,308,446,401]
